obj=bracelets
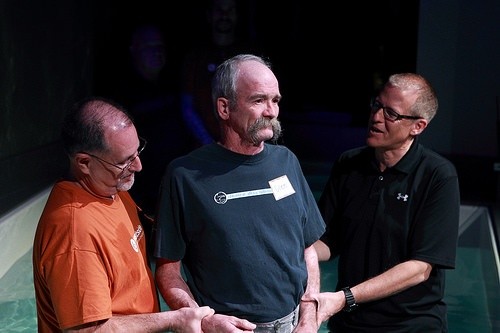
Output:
[344,287,357,312]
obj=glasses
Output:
[77,135,147,174]
[370,98,424,121]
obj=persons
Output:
[33,98,215,333]
[302,74,459,333]
[147,54,326,333]
